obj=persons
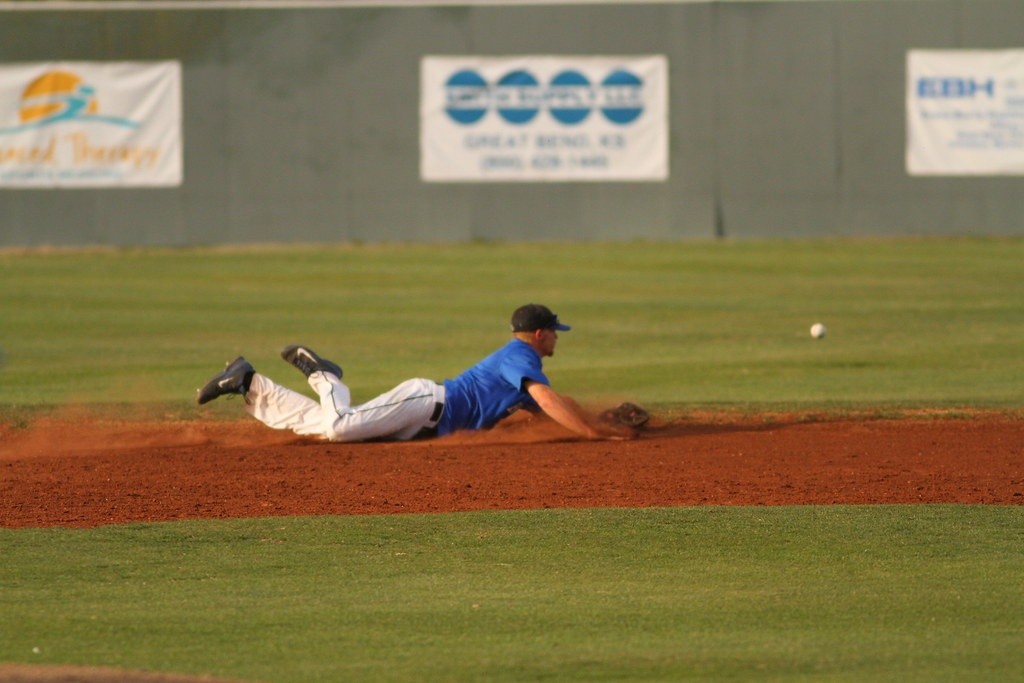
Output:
[196,304,650,442]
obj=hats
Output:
[510,304,571,332]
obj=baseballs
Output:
[809,323,827,340]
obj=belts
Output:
[421,402,444,434]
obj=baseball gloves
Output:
[598,402,650,428]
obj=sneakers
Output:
[280,345,343,380]
[195,355,255,404]
